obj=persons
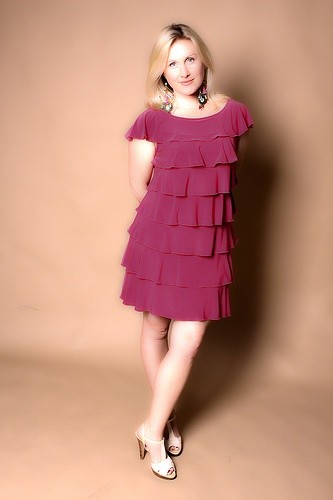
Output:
[124,22,254,481]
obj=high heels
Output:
[164,409,183,456]
[135,424,177,480]
